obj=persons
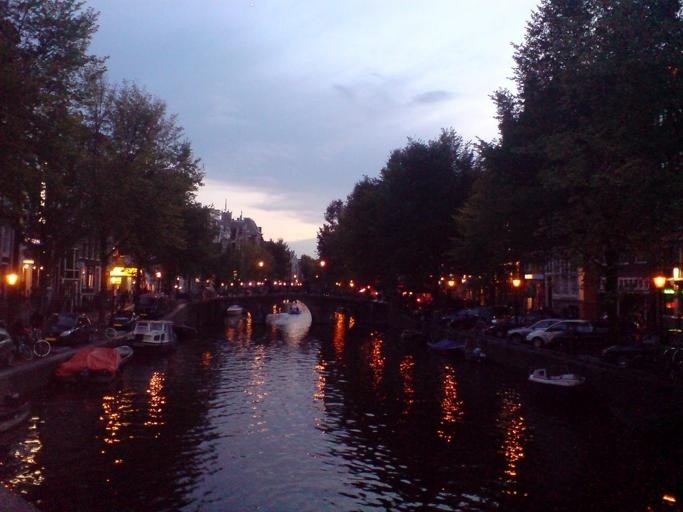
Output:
[0,288,131,358]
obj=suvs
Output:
[134,295,162,316]
[524,320,594,352]
[551,320,640,354]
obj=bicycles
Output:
[94,320,117,340]
[664,332,682,379]
[19,329,50,361]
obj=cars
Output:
[109,311,139,329]
[506,317,563,345]
[0,326,16,367]
[494,311,556,339]
[42,312,91,345]
[440,303,514,328]
[601,329,668,364]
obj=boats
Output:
[0,391,31,435]
[335,303,352,313]
[226,305,242,315]
[78,345,136,389]
[427,338,486,363]
[126,320,195,351]
[286,304,302,315]
[528,368,585,391]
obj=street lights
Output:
[6,273,18,330]
[654,268,665,337]
[448,278,455,302]
[155,271,161,294]
[513,277,521,318]
[107,275,121,315]
